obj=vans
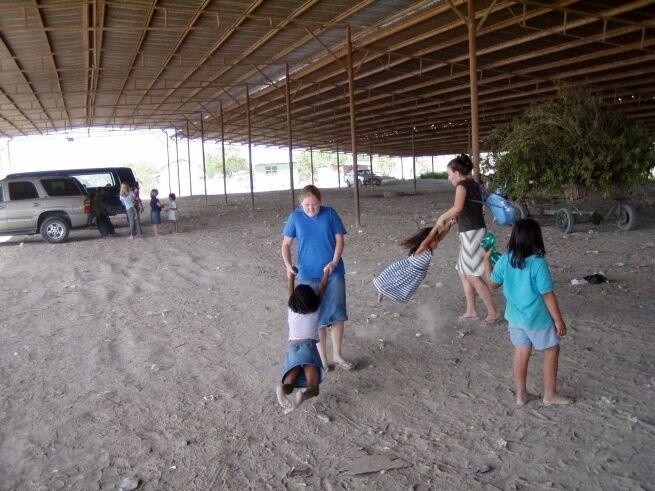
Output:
[0,167,139,244]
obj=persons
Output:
[434,154,504,328]
[149,187,166,237]
[163,192,178,234]
[280,184,357,372]
[481,216,575,407]
[92,186,120,240]
[275,265,333,413]
[130,181,145,221]
[118,181,142,241]
[373,216,456,308]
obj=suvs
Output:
[344,169,382,186]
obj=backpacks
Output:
[485,190,518,227]
